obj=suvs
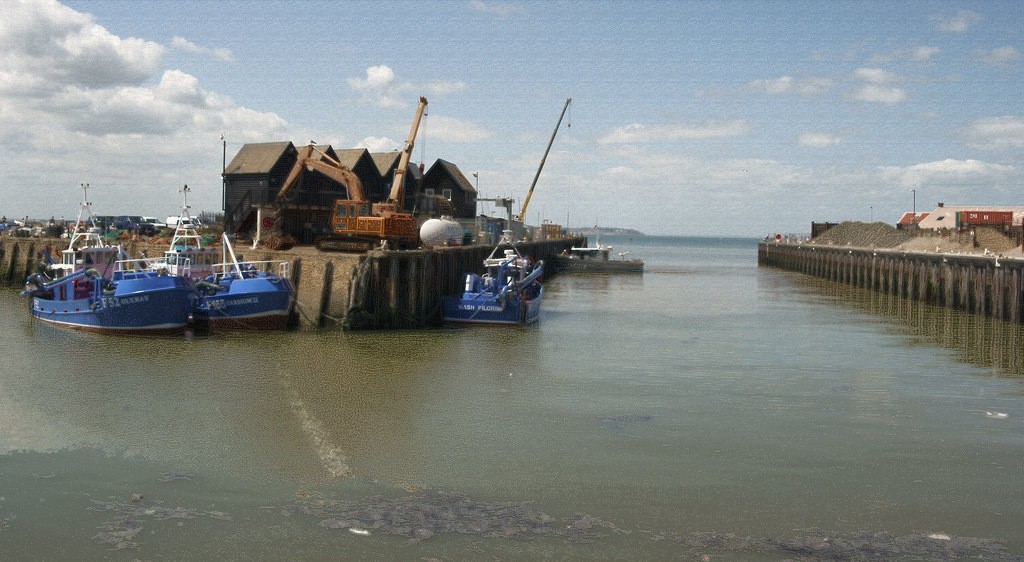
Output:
[84,213,208,238]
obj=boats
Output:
[19,180,199,340]
[552,225,645,275]
[435,197,545,328]
[153,183,294,336]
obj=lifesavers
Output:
[262,217,274,228]
[776,234,781,239]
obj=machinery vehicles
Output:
[262,96,431,253]
